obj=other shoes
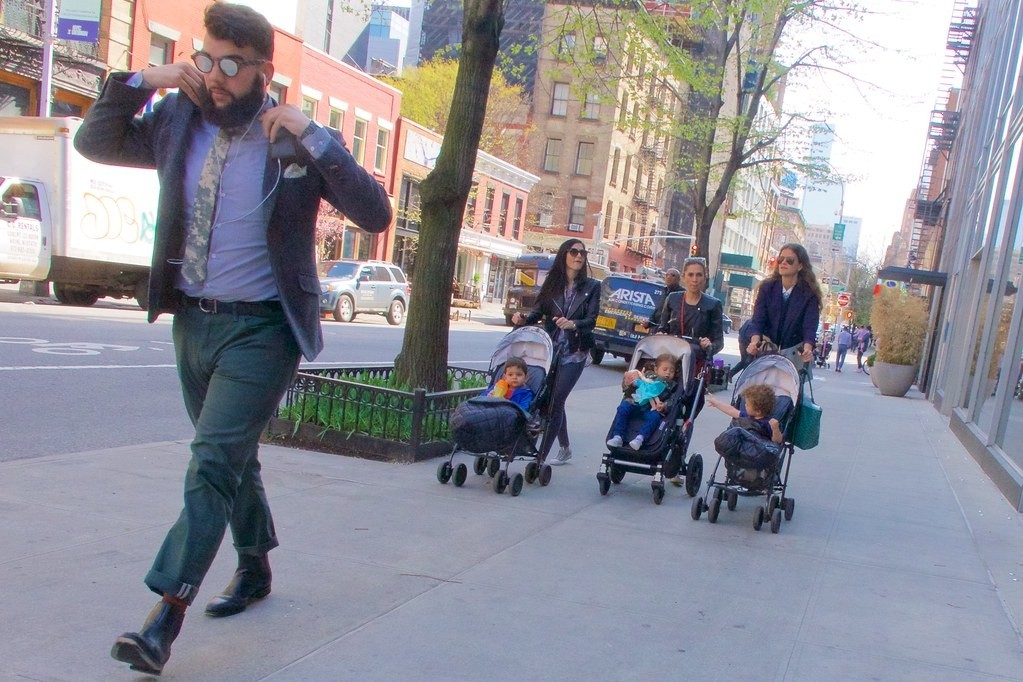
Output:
[607,435,623,448]
[669,470,685,485]
[629,438,643,451]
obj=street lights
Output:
[802,154,846,329]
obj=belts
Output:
[177,295,284,315]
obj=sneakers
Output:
[550,447,572,466]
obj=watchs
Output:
[301,120,323,140]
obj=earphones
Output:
[261,73,267,87]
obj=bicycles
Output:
[863,359,875,376]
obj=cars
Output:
[722,312,732,335]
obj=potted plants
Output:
[868,354,877,388]
[871,286,932,396]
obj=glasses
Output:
[683,257,707,265]
[777,257,797,265]
[664,273,676,278]
[567,248,589,258]
[190,49,271,78]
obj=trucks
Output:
[585,273,667,365]
[1,112,165,315]
[504,254,611,326]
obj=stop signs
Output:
[838,295,849,308]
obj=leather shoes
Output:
[110,600,186,676]
[204,552,273,617]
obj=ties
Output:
[179,128,238,285]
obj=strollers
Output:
[690,344,813,534]
[436,315,576,497]
[594,320,714,505]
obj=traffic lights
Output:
[769,255,776,266]
[690,245,698,259]
[846,311,854,319]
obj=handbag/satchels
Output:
[786,369,822,450]
[694,360,710,387]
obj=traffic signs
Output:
[829,246,841,252]
[822,277,839,285]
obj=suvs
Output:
[316,260,408,326]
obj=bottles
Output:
[493,379,510,399]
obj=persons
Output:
[853,324,871,373]
[707,385,783,443]
[625,367,667,411]
[480,357,534,412]
[835,327,851,372]
[607,354,677,450]
[511,238,601,465]
[728,319,753,384]
[746,243,823,362]
[641,268,685,329]
[657,257,724,484]
[73,0,393,676]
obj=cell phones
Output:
[269,128,297,161]
